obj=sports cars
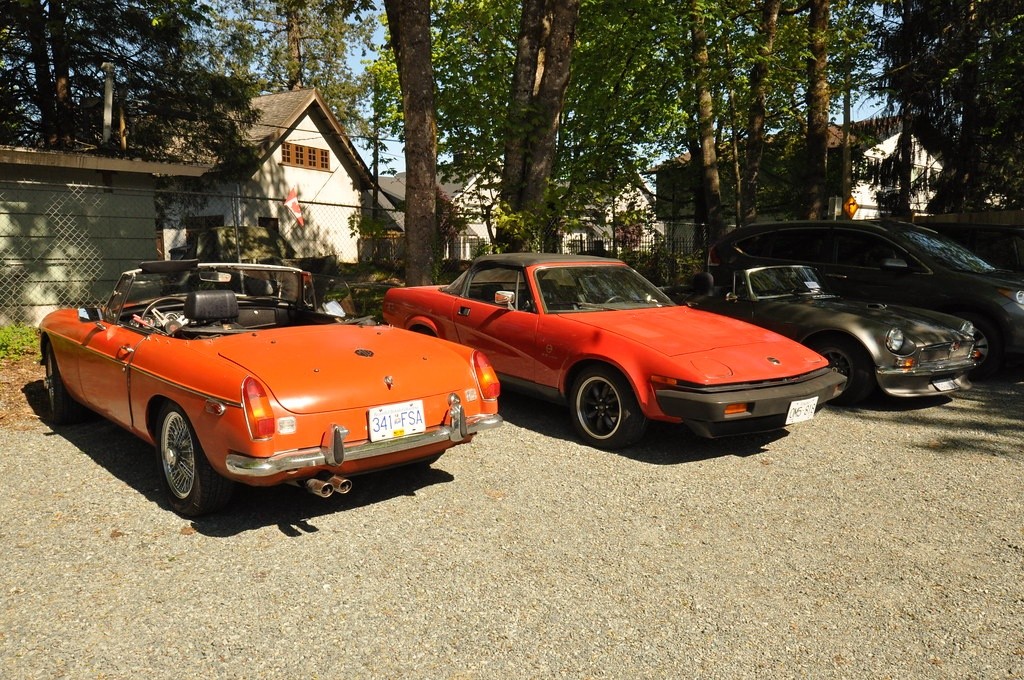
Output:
[638,264,982,405]
[382,252,849,452]
[36,259,506,519]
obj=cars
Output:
[905,207,1024,269]
[707,219,1024,381]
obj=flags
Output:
[283,189,304,227]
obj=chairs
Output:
[480,279,561,312]
[174,289,240,338]
